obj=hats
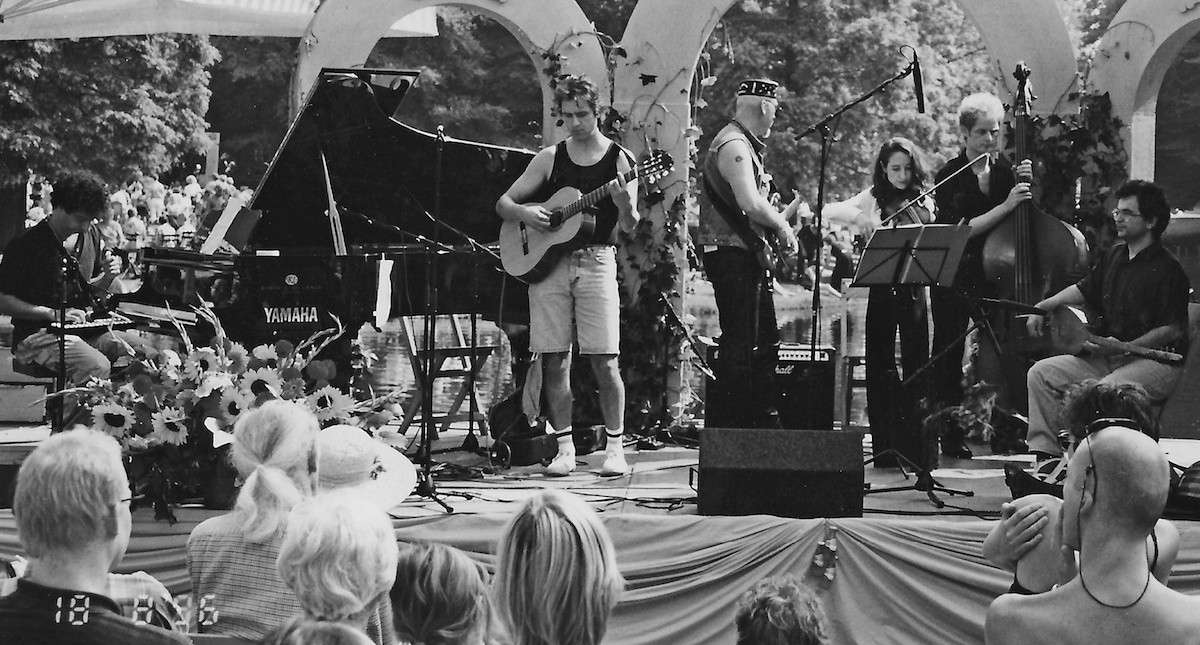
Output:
[315,423,417,514]
[737,79,779,98]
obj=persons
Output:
[1026,178,1192,484]
[930,91,1033,457]
[733,572,835,645]
[984,377,1200,644]
[696,76,936,468]
[0,163,630,645]
[495,73,640,478]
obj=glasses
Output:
[1057,432,1078,451]
[1084,418,1142,505]
[1111,208,1141,222]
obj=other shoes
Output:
[941,434,973,459]
[601,450,628,476]
[546,449,577,476]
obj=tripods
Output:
[408,138,472,514]
[850,222,979,505]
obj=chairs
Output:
[835,274,904,430]
[1150,300,1200,423]
[398,316,499,440]
[12,358,58,433]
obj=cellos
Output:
[966,57,1089,400]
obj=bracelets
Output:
[1123,342,1130,357]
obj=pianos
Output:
[94,65,541,466]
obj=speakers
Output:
[705,342,847,429]
[697,426,864,519]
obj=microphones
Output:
[913,51,925,113]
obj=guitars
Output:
[499,150,675,284]
[757,171,797,280]
[1050,300,1183,362]
